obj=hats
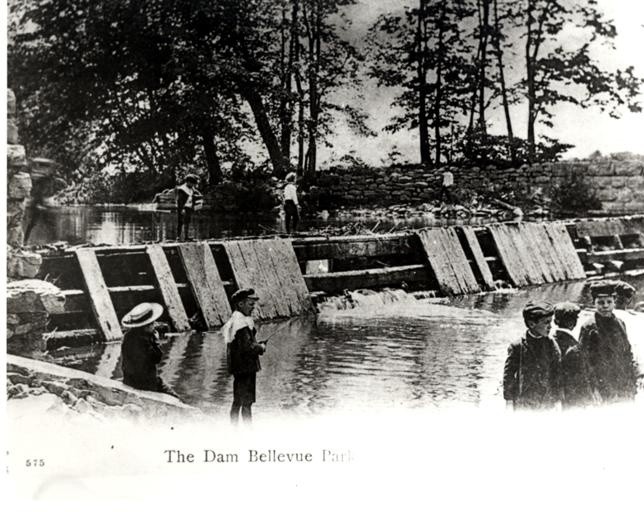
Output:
[555,302,580,315]
[187,176,197,184]
[522,300,554,320]
[121,301,164,328]
[231,287,259,303]
[591,282,617,297]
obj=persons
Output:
[23,169,68,244]
[553,302,598,412]
[504,301,566,412]
[284,173,301,237]
[176,175,201,241]
[434,165,454,207]
[121,301,183,400]
[580,286,644,403]
[225,288,266,422]
